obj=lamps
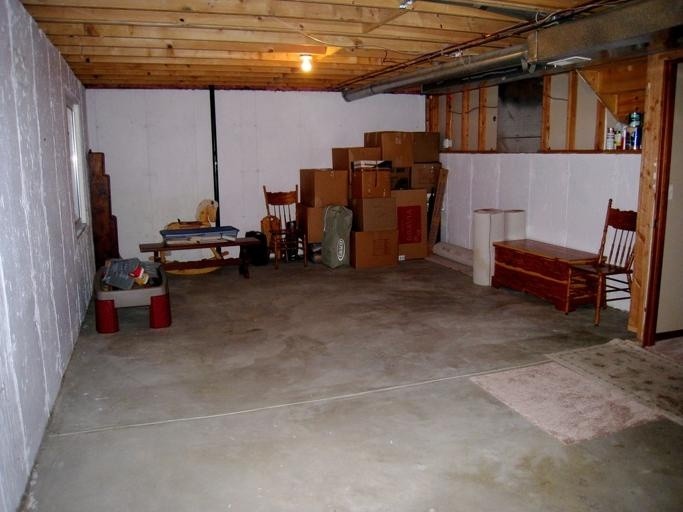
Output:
[300,55,312,71]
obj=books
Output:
[162,233,240,246]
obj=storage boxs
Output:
[299,131,441,270]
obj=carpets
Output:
[545,337,682,426]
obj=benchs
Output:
[140,237,260,279]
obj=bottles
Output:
[605,112,641,151]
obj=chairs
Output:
[565,199,637,325]
[264,185,308,269]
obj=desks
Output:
[94,266,172,333]
[491,239,607,314]
